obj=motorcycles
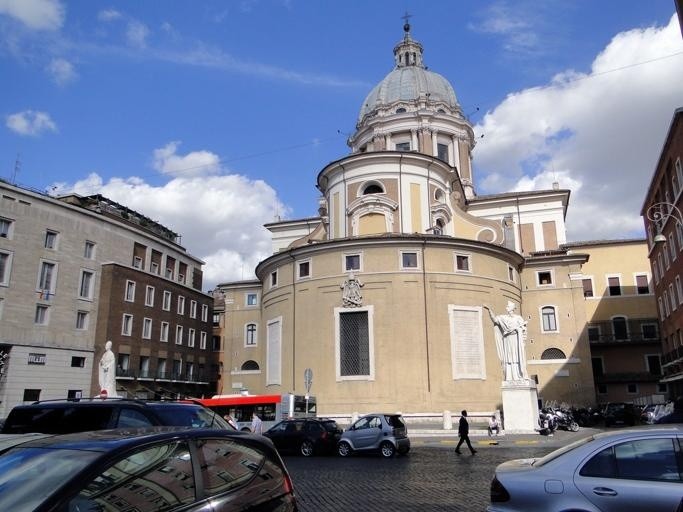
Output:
[539,399,580,435]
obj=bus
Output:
[173,393,317,436]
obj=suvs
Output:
[0,396,242,434]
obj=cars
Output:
[603,402,635,427]
[336,413,411,458]
[262,418,343,458]
[484,427,683,512]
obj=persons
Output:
[455,410,477,454]
[251,412,263,436]
[483,300,528,380]
[98,341,118,398]
[487,415,499,437]
[223,415,238,430]
